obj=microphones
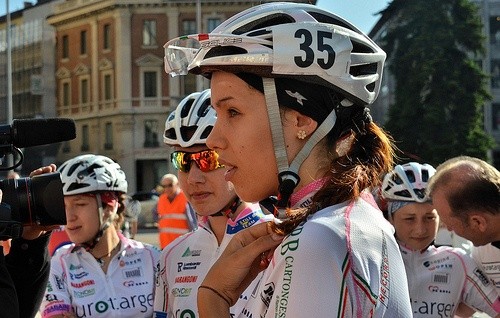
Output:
[0,119,77,148]
